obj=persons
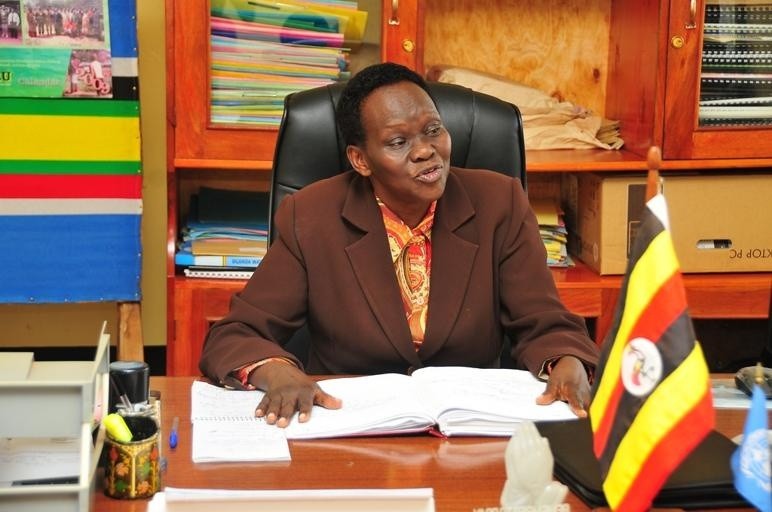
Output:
[0,3,21,40]
[198,62,602,429]
[27,5,105,42]
[63,50,114,93]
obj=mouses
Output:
[734,366,772,400]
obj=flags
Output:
[729,382,772,512]
[588,192,718,512]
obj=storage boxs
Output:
[559,174,772,277]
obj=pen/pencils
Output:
[110,371,133,411]
[169,416,180,448]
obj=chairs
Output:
[266,76,526,376]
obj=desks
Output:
[91,362,767,512]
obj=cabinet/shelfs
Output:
[607,0,772,161]
[174,0,664,163]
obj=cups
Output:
[103,415,159,500]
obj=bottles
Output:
[109,361,150,415]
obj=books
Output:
[699,0,771,126]
[533,418,750,510]
[208,1,369,126]
[280,365,582,441]
[176,207,271,279]
